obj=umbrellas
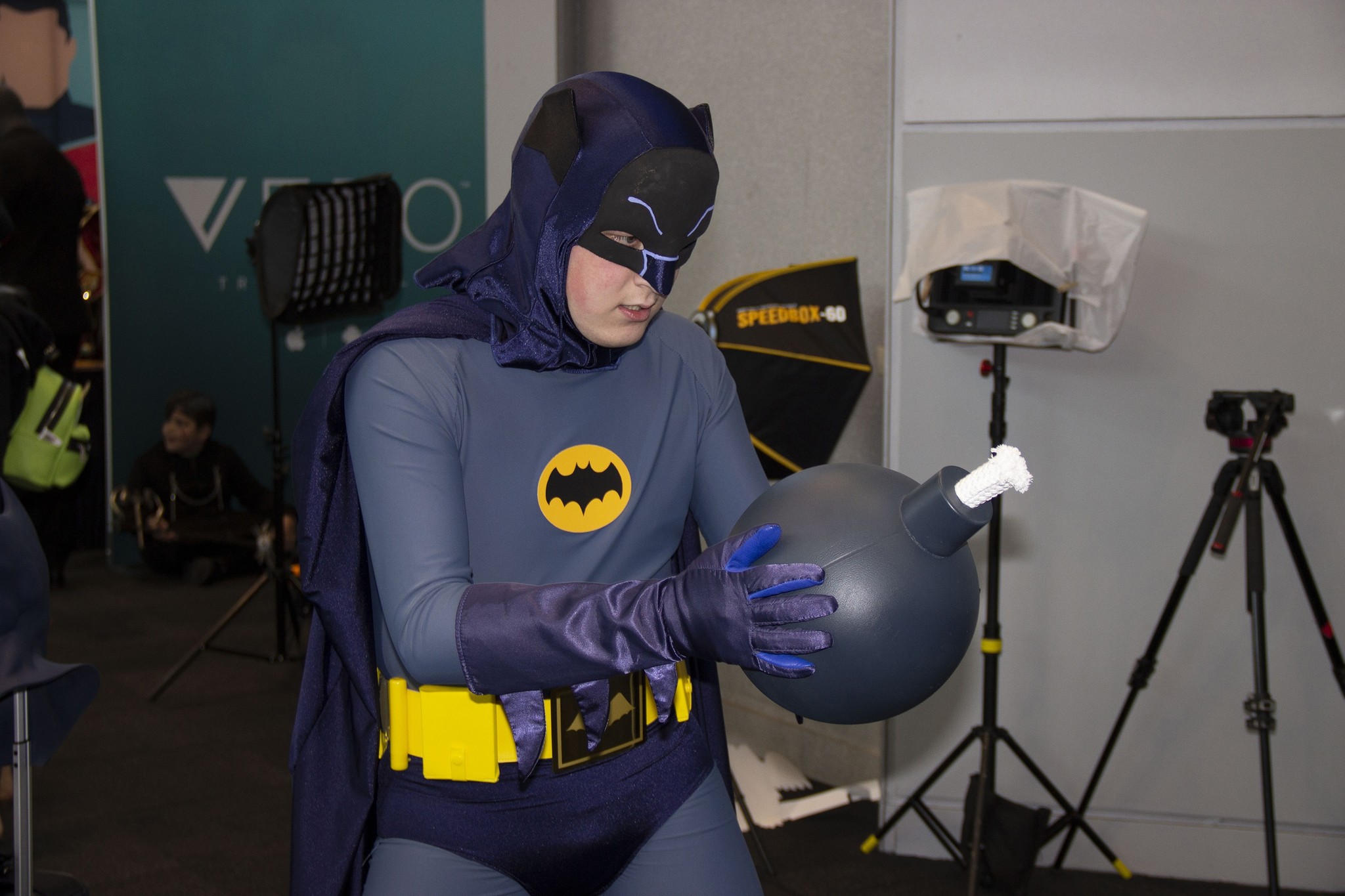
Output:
[687,258,871,477]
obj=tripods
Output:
[151,319,310,705]
[858,343,1345,896]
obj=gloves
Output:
[454,522,838,793]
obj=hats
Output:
[166,390,215,427]
[0,478,101,775]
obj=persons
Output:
[0,90,90,589]
[110,390,278,585]
[298,69,838,896]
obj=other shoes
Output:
[187,557,213,586]
